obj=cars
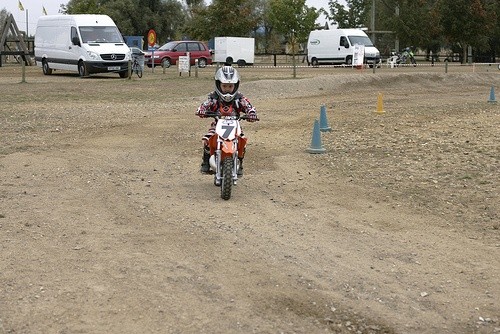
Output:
[144,41,212,69]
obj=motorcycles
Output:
[390,46,417,67]
[196,110,259,200]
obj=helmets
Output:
[214,66,240,102]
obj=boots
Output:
[201,149,210,172]
[237,156,243,176]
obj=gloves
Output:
[245,110,256,122]
[197,108,205,118]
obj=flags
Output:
[18,0,24,10]
[43,7,47,15]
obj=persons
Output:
[195,66,258,177]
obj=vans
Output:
[307,28,380,68]
[34,14,131,79]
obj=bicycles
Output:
[131,52,144,78]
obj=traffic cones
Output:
[319,106,332,131]
[306,119,327,153]
[487,83,497,102]
[373,93,386,113]
